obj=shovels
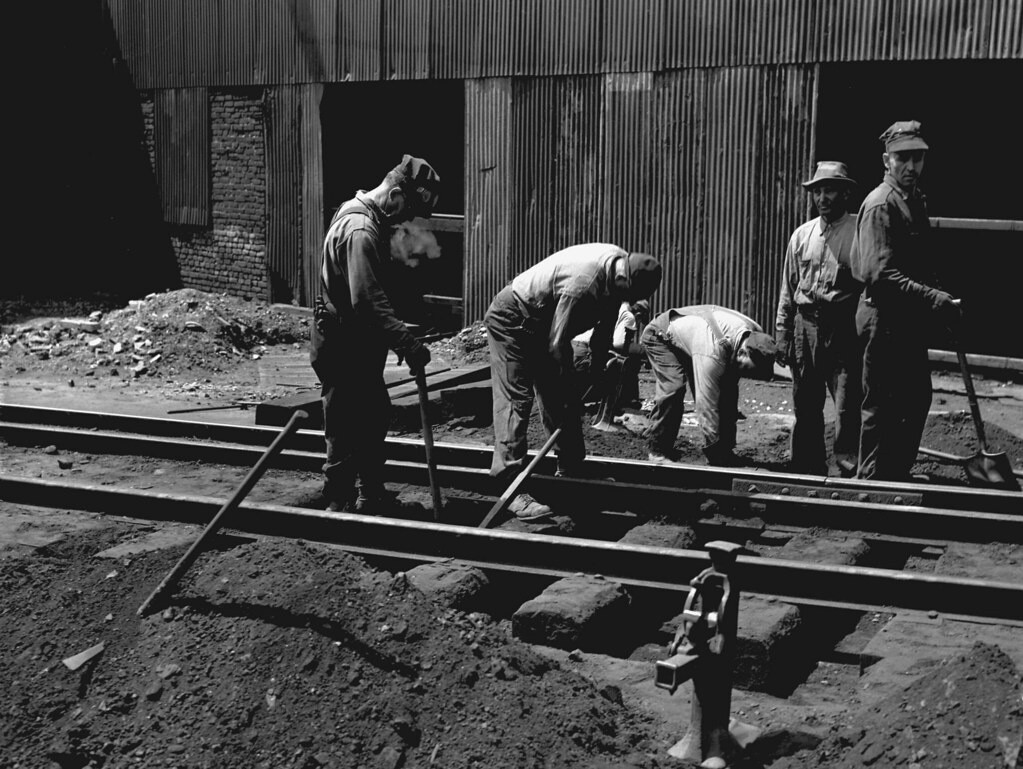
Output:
[939,298,1023,492]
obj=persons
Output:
[850,121,964,482]
[572,299,650,405]
[775,161,860,475]
[640,305,778,468]
[310,156,443,514]
[485,244,663,522]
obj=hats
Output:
[624,252,662,301]
[396,154,440,219]
[801,161,858,192]
[747,331,779,380]
[878,120,928,153]
[636,300,651,326]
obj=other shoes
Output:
[505,492,553,520]
[324,499,345,511]
[556,469,616,481]
[356,493,424,516]
[649,452,673,461]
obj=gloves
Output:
[586,365,608,391]
[774,330,792,368]
[551,345,576,375]
[404,344,431,376]
[930,289,963,325]
